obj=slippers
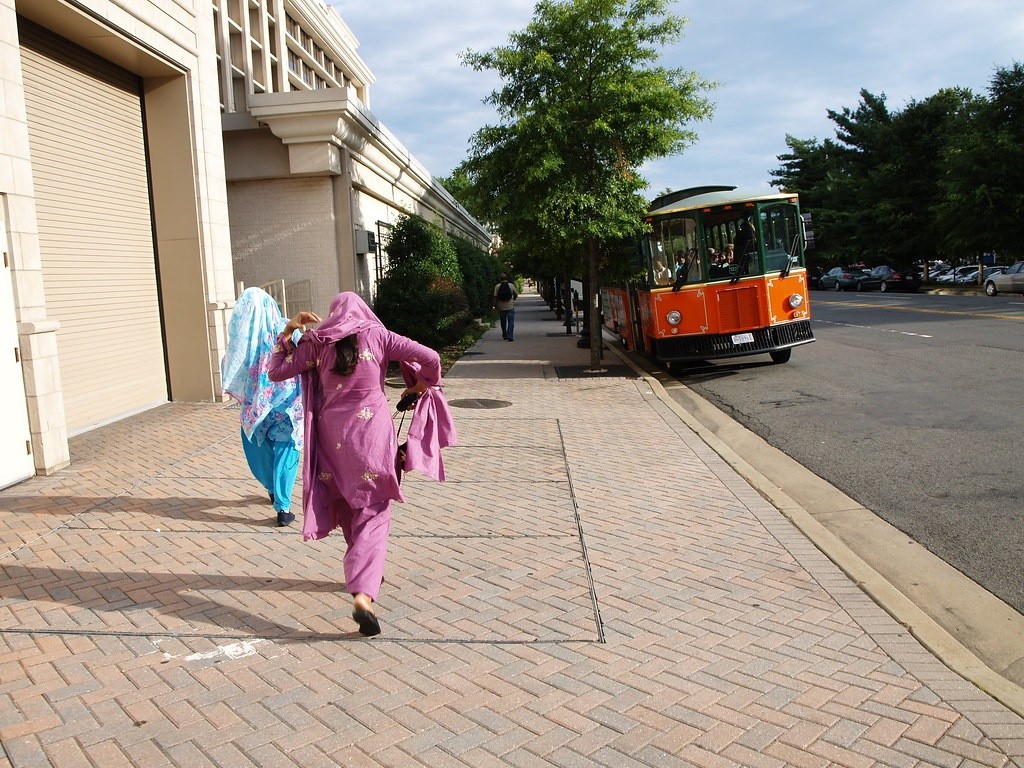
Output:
[353,610,381,635]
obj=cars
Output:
[983,261,1024,296]
[920,265,1008,284]
[818,267,864,291]
[856,264,922,293]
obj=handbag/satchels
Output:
[398,442,408,471]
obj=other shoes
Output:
[277,510,295,526]
[504,336,508,340]
[269,493,275,504]
[508,338,513,341]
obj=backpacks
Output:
[497,282,513,302]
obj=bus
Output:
[600,185,816,377]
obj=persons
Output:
[220,287,304,527]
[492,273,518,341]
[672,244,734,279]
[652,256,672,285]
[268,292,458,637]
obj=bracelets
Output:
[492,306,495,309]
